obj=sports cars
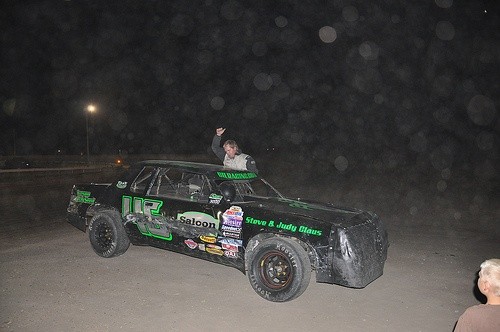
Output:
[66,160,389,305]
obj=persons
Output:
[452,258,499,331]
[211,126,258,172]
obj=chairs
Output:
[188,177,206,201]
